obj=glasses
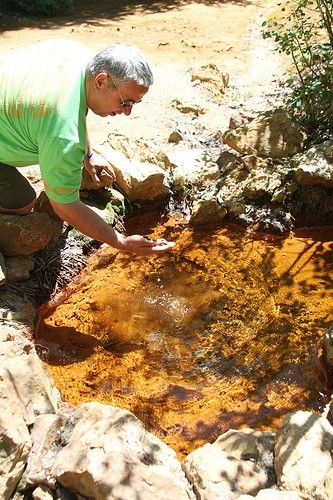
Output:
[107,73,142,108]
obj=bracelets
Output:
[84,151,94,160]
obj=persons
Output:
[0,40,176,256]
